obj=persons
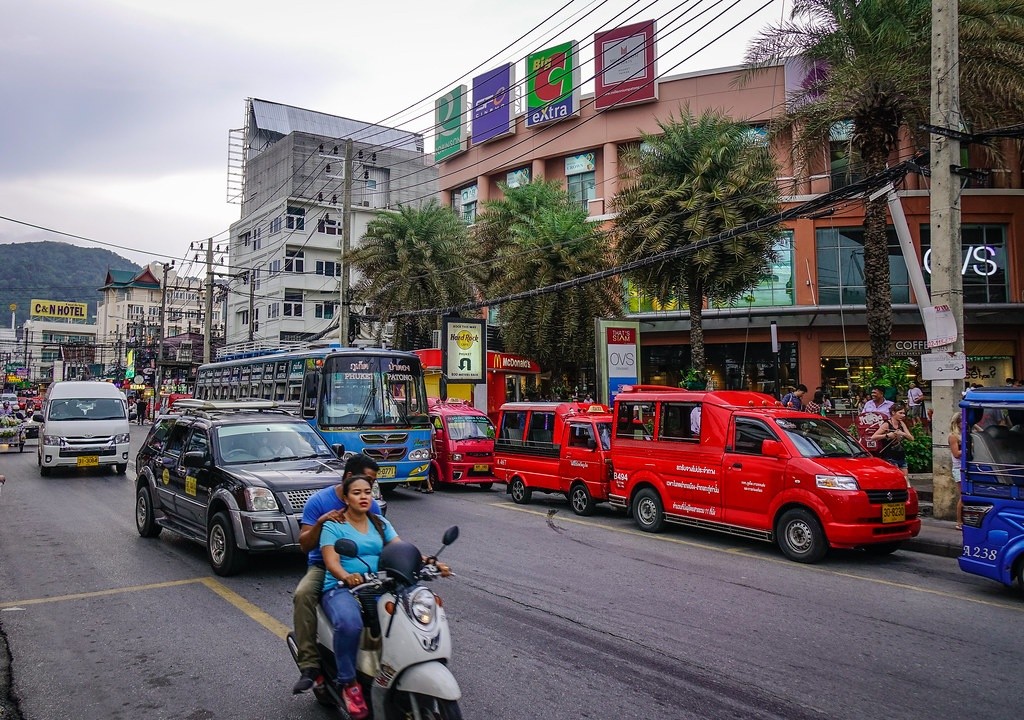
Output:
[319,475,452,719]
[257,434,294,457]
[964,378,1024,432]
[508,385,594,404]
[290,453,382,696]
[0,395,45,437]
[870,403,914,496]
[51,402,73,418]
[851,382,932,437]
[782,383,832,416]
[128,395,147,425]
[948,411,973,531]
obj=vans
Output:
[492,401,654,516]
[33,381,137,478]
[607,384,920,563]
[957,387,1024,594]
[0,394,19,406]
[390,397,504,490]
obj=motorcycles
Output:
[26,406,34,418]
[285,524,464,720]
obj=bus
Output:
[192,346,450,496]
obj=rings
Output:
[354,578,359,582]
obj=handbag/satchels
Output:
[912,398,921,404]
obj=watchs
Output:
[885,433,889,439]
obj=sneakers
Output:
[293,667,324,695]
[341,682,369,720]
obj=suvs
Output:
[135,393,388,577]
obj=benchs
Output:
[970,423,1024,484]
